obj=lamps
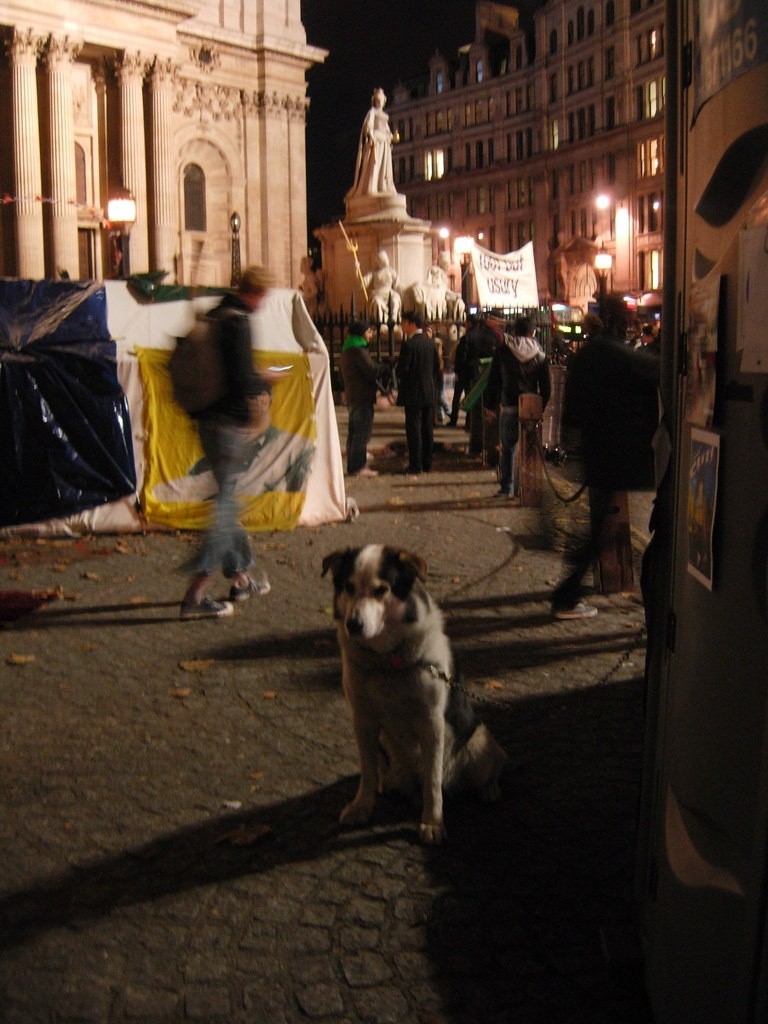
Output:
[105,184,138,230]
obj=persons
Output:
[343,88,400,200]
[180,267,286,619]
[421,294,661,621]
[299,250,466,326]
[340,322,384,477]
[395,310,443,474]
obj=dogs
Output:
[317,544,497,841]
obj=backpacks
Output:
[166,312,222,409]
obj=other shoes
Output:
[179,597,233,619]
[367,452,373,460]
[494,490,511,498]
[229,577,271,600]
[354,468,378,477]
[553,602,598,619]
